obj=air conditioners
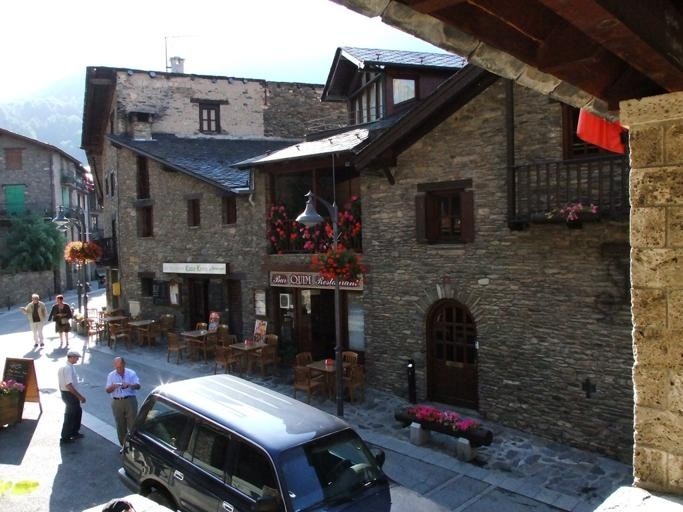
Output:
[279,293,292,308]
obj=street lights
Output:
[294,191,342,419]
[51,206,87,328]
[57,224,83,322]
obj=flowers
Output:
[0,379,26,398]
[63,241,102,263]
[265,194,366,287]
[406,404,483,433]
[545,199,597,223]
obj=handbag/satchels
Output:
[61,318,68,324]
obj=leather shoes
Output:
[64,436,75,442]
[35,344,38,347]
[40,343,44,346]
[75,434,84,439]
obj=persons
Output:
[48,295,72,346]
[19,293,48,347]
[58,351,86,440]
[105,356,141,454]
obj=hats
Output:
[67,351,81,358]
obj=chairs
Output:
[167,333,187,365]
[85,320,99,344]
[153,315,176,342]
[137,319,163,349]
[192,332,216,364]
[108,325,131,351]
[296,352,325,384]
[333,352,358,381]
[128,315,142,333]
[206,325,228,341]
[248,343,277,376]
[255,334,278,357]
[97,312,110,340]
[291,366,324,405]
[183,322,207,354]
[211,345,242,378]
[221,335,242,365]
[332,365,365,406]
[110,309,124,316]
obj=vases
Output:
[393,406,492,449]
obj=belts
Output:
[113,395,131,400]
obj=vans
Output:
[117,374,391,512]
